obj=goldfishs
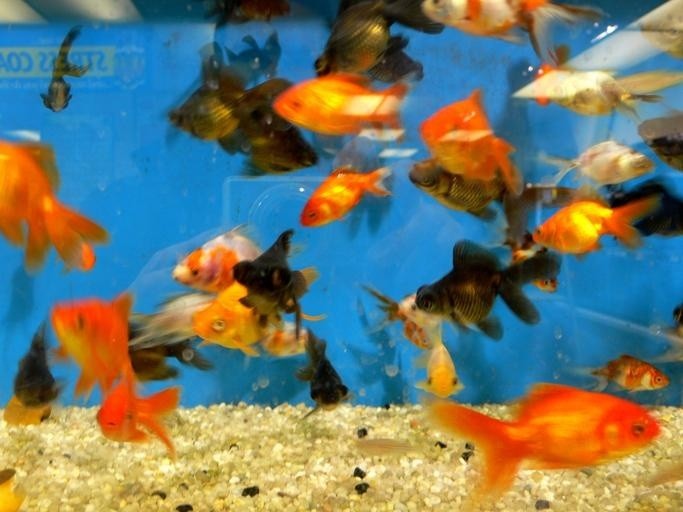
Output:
[0,1,682,512]
